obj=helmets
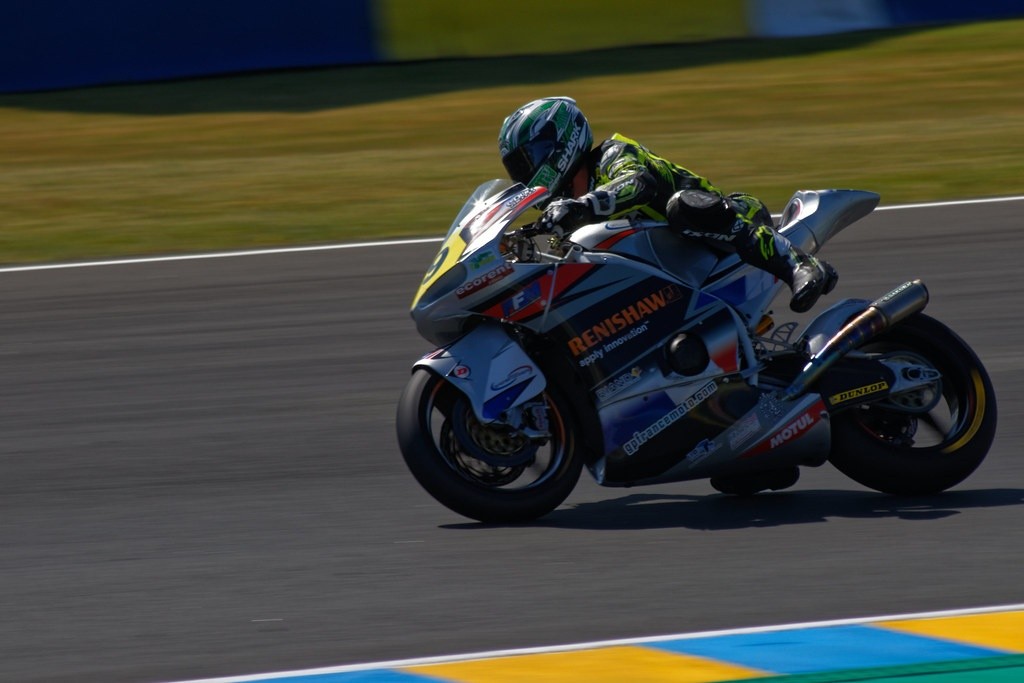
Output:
[498,95,594,212]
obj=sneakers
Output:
[789,245,839,313]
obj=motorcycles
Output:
[396,179,998,524]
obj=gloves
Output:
[538,189,619,242]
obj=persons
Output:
[498,96,838,494]
